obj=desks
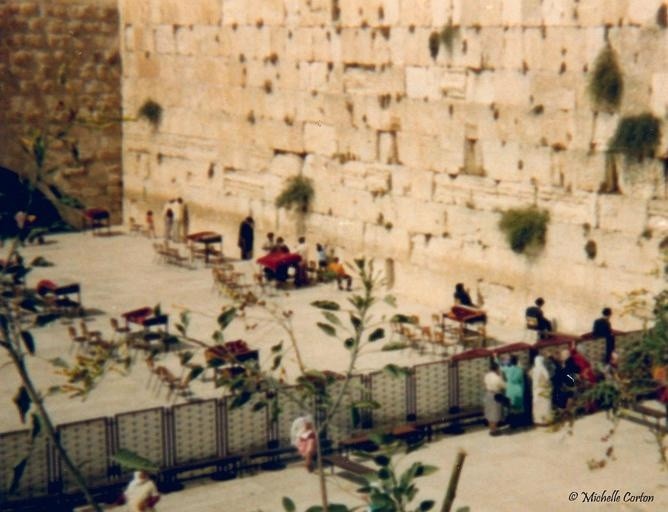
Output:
[122,308,169,351]
[207,340,259,387]
[256,252,301,288]
[83,209,110,236]
[39,276,81,315]
[442,309,487,336]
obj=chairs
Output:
[130,218,271,304]
[307,261,339,291]
[524,317,539,345]
[403,314,488,358]
[67,318,204,406]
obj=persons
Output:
[453,282,475,307]
[124,471,160,512]
[591,307,616,363]
[525,297,553,340]
[603,351,621,381]
[237,216,254,260]
[261,232,353,292]
[164,197,187,244]
[163,199,176,241]
[290,413,317,474]
[481,347,599,436]
[145,210,158,239]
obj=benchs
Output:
[152,406,486,494]
